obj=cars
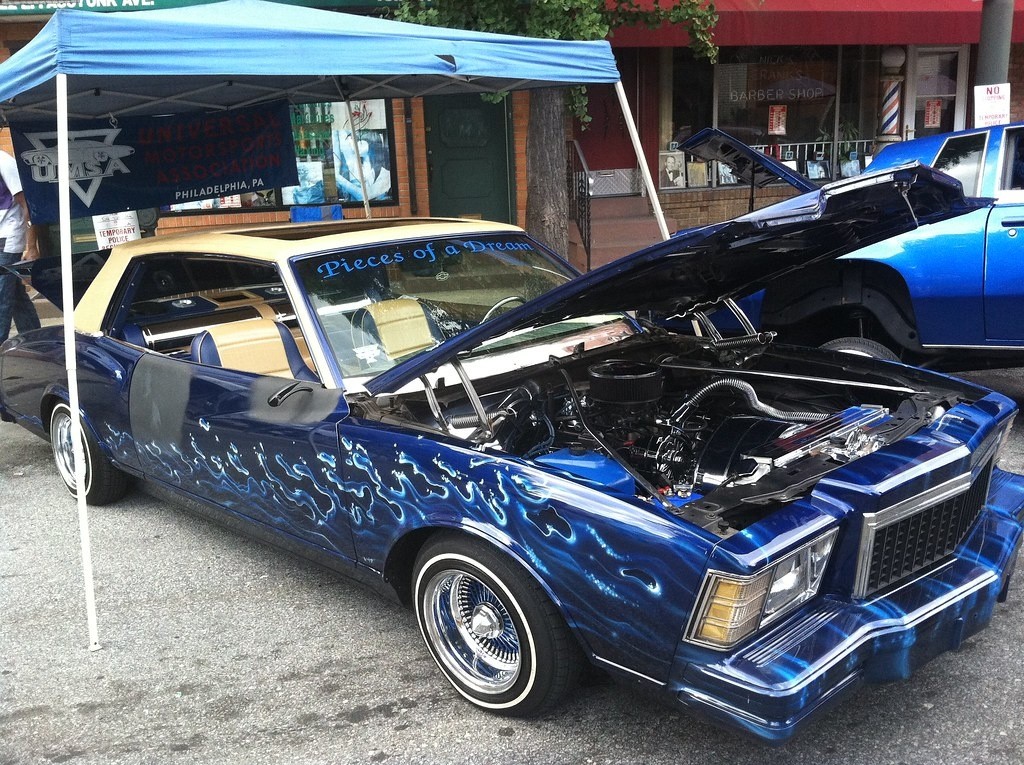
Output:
[0,161,1024,747]
[649,120,1023,371]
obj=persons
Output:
[0,151,40,345]
[659,156,683,187]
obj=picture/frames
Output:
[660,151,685,189]
[807,160,831,179]
[687,160,708,188]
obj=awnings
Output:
[602,0,1024,47]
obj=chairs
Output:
[288,203,346,224]
[351,299,445,371]
[191,319,318,382]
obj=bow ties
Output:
[668,168,672,173]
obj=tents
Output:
[0,0,672,653]
